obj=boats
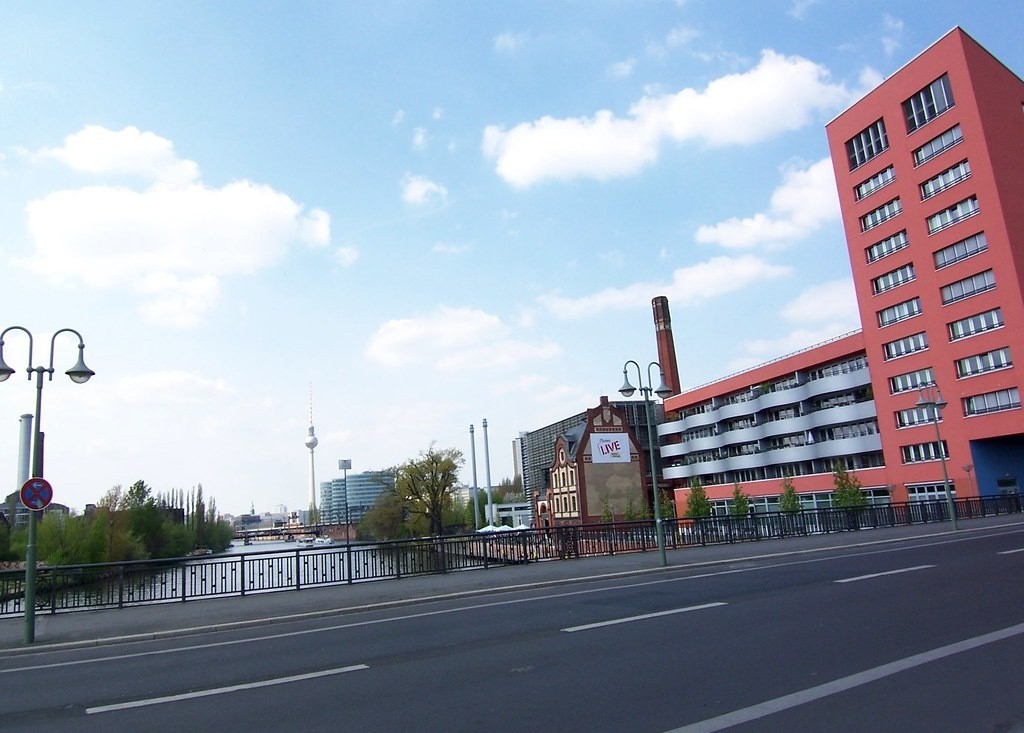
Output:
[296,537,314,548]
[313,535,332,545]
[194,543,213,556]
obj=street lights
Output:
[0,326,96,643]
[618,360,672,566]
[915,380,958,532]
